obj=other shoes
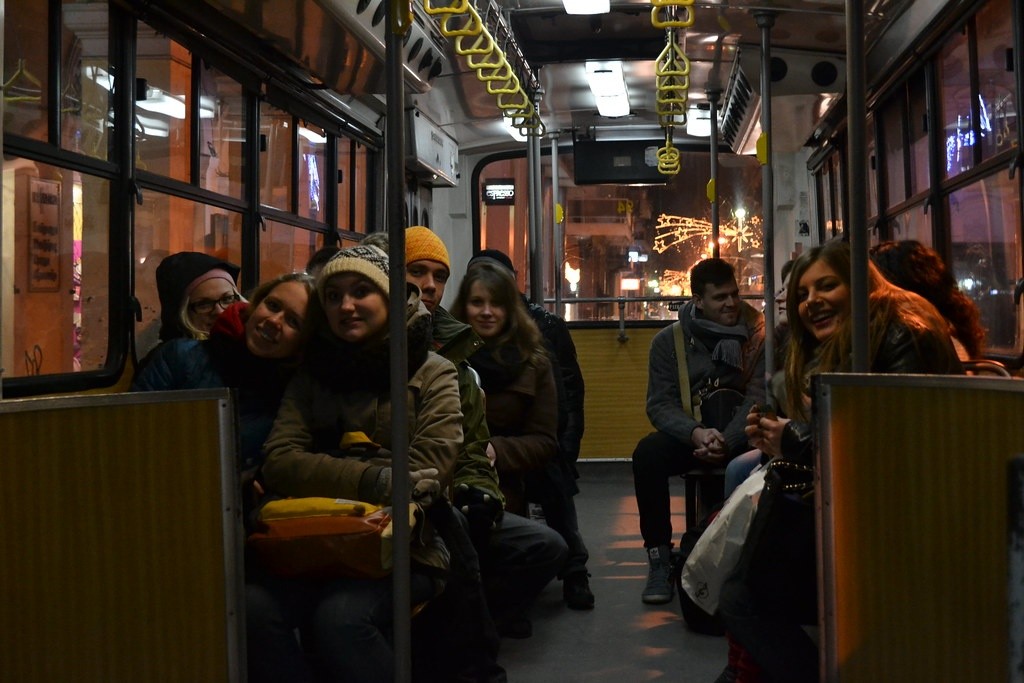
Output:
[495,584,532,638]
[560,563,595,608]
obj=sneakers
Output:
[640,543,673,603]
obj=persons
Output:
[632,237,1024,683]
[128,224,595,683]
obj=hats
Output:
[405,226,451,272]
[188,269,236,292]
[466,249,516,281]
[317,233,389,304]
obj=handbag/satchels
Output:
[735,462,817,628]
[243,497,420,578]
[679,454,777,613]
[694,388,744,432]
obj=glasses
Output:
[187,294,237,313]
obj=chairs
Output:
[680,467,724,531]
[962,358,1012,378]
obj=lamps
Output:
[584,57,630,118]
[562,0,611,15]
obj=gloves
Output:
[368,467,441,507]
[449,483,504,531]
